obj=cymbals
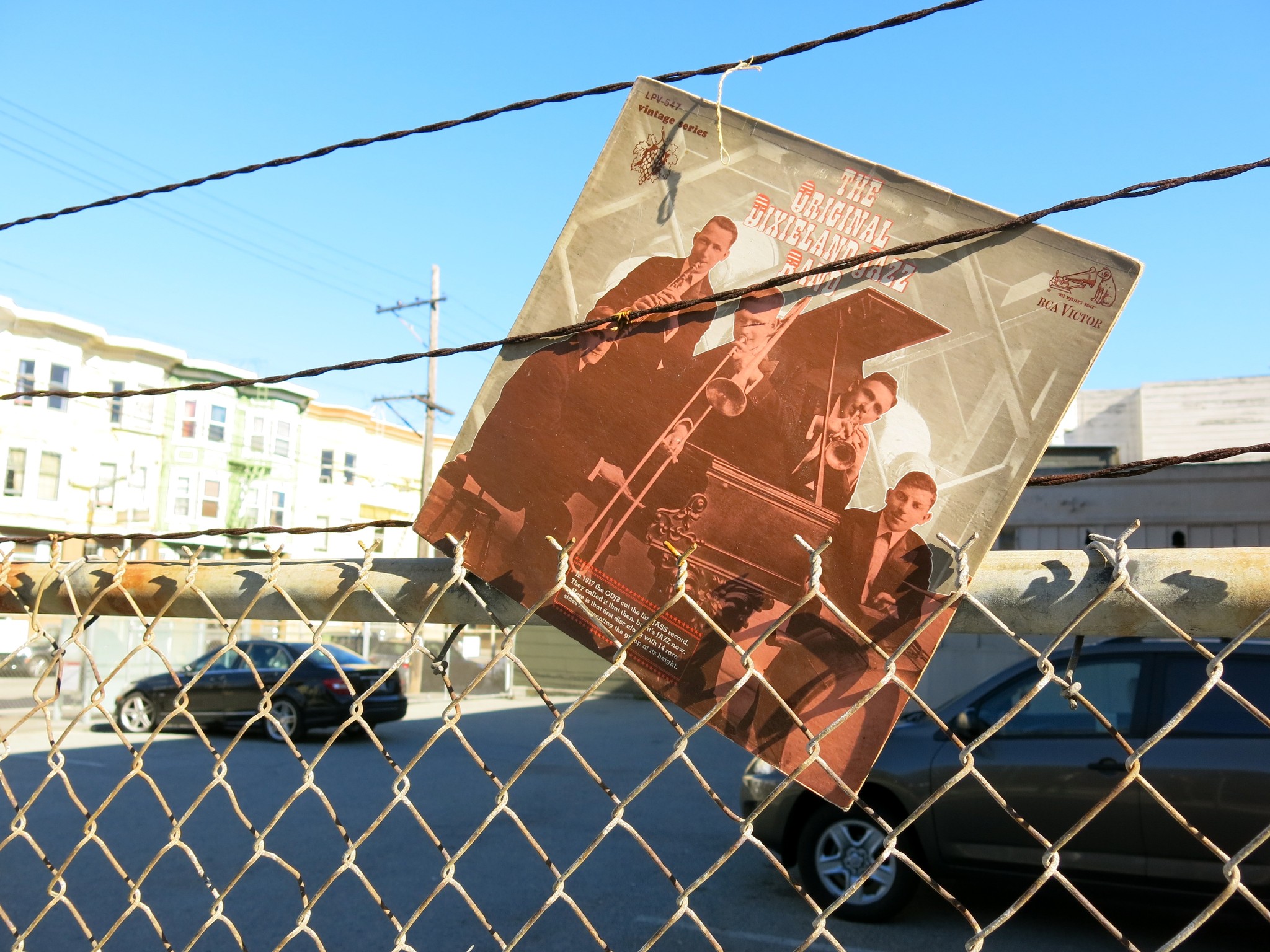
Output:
[901,578,961,611]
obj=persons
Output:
[666,281,801,489]
[776,469,940,654]
[595,211,737,425]
[465,304,647,601]
[782,369,899,515]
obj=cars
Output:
[740,638,1270,925]
[114,640,407,743]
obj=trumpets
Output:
[823,410,863,473]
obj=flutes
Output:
[610,260,704,352]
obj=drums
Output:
[755,623,922,807]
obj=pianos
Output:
[580,435,847,619]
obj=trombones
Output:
[567,293,814,577]
